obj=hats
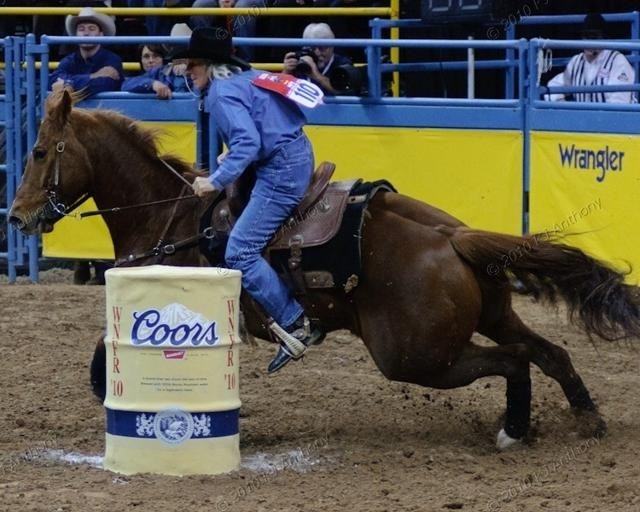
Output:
[66,8,117,36]
[568,13,613,37]
[163,23,193,50]
[171,27,251,68]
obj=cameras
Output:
[287,46,318,80]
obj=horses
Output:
[6,85,640,453]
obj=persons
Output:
[537,12,638,103]
[172,25,325,373]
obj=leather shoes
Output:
[269,316,321,373]
